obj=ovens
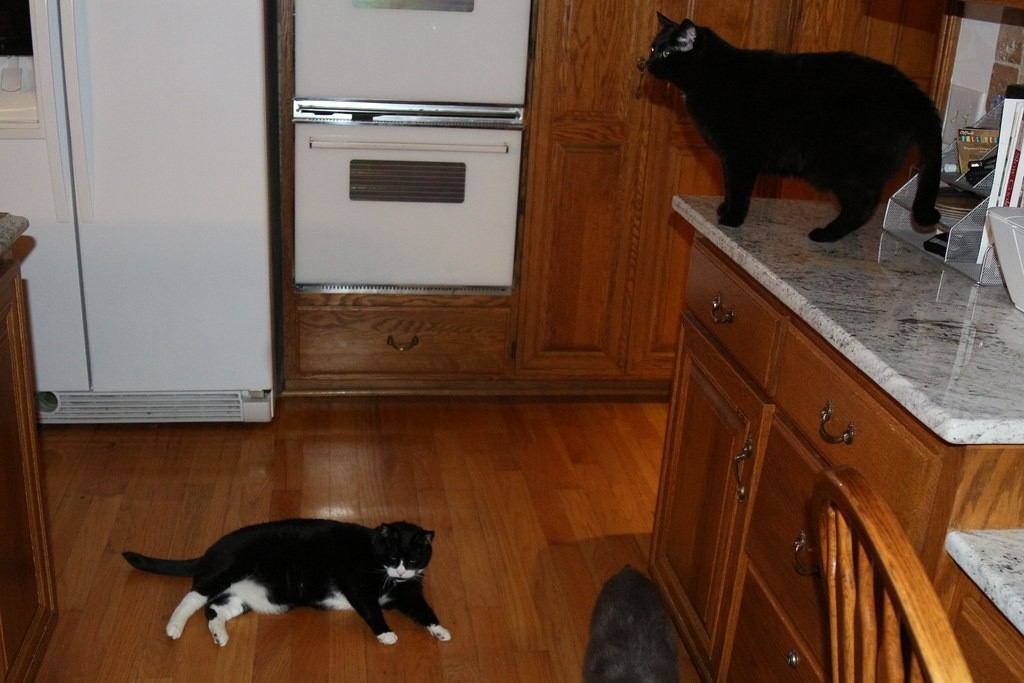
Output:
[293,0,532,297]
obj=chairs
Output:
[810,465,973,683]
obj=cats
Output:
[121,518,450,647]
[647,10,942,243]
[582,565,680,683]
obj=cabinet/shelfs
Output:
[281,294,517,394]
[947,567,1023,683]
[2,235,61,682]
[647,229,964,683]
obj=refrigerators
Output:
[0,0,280,425]
